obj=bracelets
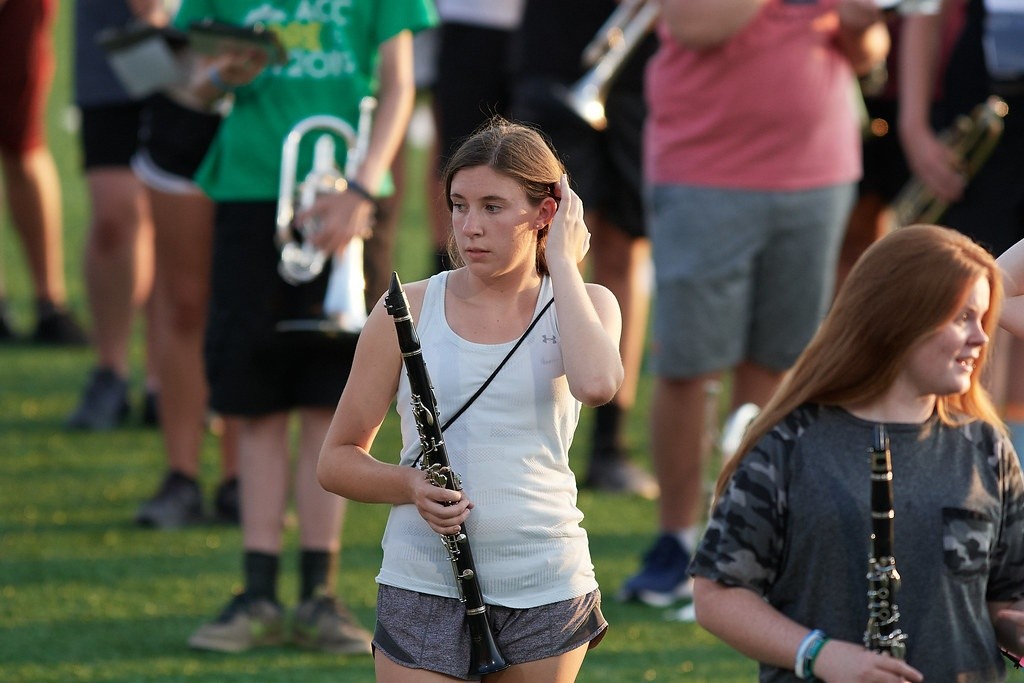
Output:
[803,636,830,683]
[207,62,242,96]
[346,180,380,211]
[794,629,825,679]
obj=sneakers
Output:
[613,528,698,613]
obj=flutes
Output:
[383,271,513,677]
[862,425,909,662]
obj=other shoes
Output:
[187,588,292,653]
[67,365,132,436]
[288,582,376,655]
[0,294,90,344]
[214,475,242,529]
[584,448,665,502]
[135,467,203,529]
[139,390,161,431]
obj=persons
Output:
[175,1,438,659]
[681,224,1023,683]
[311,115,622,683]
[70,3,1023,525]
[624,0,891,610]
[1,0,82,343]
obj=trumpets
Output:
[886,94,1009,229]
[548,0,664,131]
[275,97,378,336]
